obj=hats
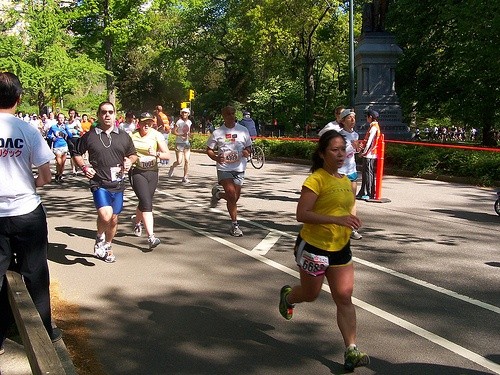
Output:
[339,109,356,121]
[180,108,190,116]
[365,110,379,119]
[139,112,154,122]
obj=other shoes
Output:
[370,194,375,199]
[55,175,63,182]
[169,166,174,177]
[356,194,364,198]
[52,328,62,343]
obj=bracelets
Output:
[80,165,89,172]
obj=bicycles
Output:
[494,191,500,218]
[247,138,265,170]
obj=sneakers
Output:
[148,236,160,248]
[278,285,295,320]
[229,224,243,237]
[344,347,369,370]
[94,234,106,259]
[211,187,220,208]
[131,215,142,237]
[182,177,189,183]
[104,245,116,262]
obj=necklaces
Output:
[99,128,112,148]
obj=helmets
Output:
[243,112,250,116]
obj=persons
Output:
[254,115,278,138]
[205,105,253,236]
[14,105,192,185]
[0,70,63,355]
[294,120,317,139]
[334,108,363,241]
[238,110,257,162]
[354,109,380,200]
[416,125,479,142]
[318,105,348,137]
[279,126,372,371]
[121,111,171,250]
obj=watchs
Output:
[156,152,161,158]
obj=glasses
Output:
[140,122,151,127]
[100,110,114,114]
